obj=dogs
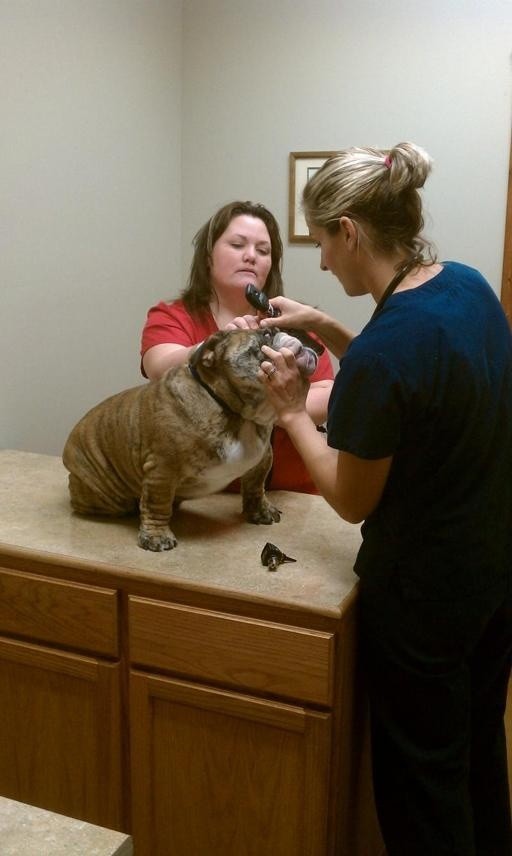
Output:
[62,324,319,552]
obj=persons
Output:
[138,197,335,493]
[256,137,512,854]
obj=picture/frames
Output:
[289,151,344,243]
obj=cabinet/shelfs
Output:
[0,554,386,856]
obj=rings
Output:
[266,366,277,377]
